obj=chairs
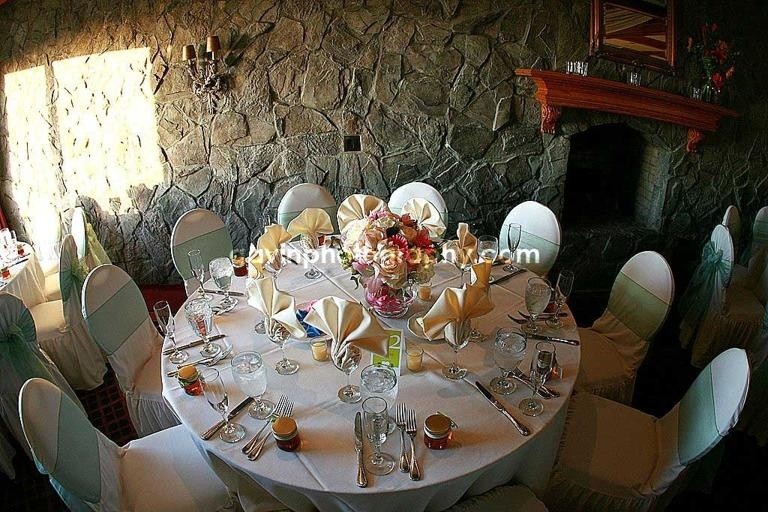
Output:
[0,174,767,511]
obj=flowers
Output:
[683,22,745,86]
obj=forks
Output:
[499,364,550,399]
[504,308,568,325]
[395,401,422,482]
[167,345,233,377]
[238,394,294,460]
[511,362,563,398]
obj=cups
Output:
[233,256,248,277]
[422,414,452,450]
[415,281,433,301]
[272,417,302,450]
[0,227,24,279]
[307,335,329,363]
[176,367,202,398]
[405,347,424,373]
[320,233,326,245]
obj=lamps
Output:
[179,32,247,96]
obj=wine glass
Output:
[334,347,362,403]
[460,273,489,343]
[252,235,320,335]
[515,341,558,417]
[522,275,551,337]
[476,234,500,285]
[546,270,576,328]
[440,319,469,380]
[198,367,247,445]
[358,364,398,478]
[267,321,300,376]
[503,222,521,271]
[152,249,235,360]
[490,325,528,395]
[230,350,276,420]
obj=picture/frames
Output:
[587,4,683,76]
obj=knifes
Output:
[528,331,580,347]
[473,378,531,438]
[489,268,527,285]
[198,288,244,296]
[202,396,253,440]
[351,410,369,489]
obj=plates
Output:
[406,308,449,342]
[289,331,333,343]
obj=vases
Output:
[700,79,733,109]
